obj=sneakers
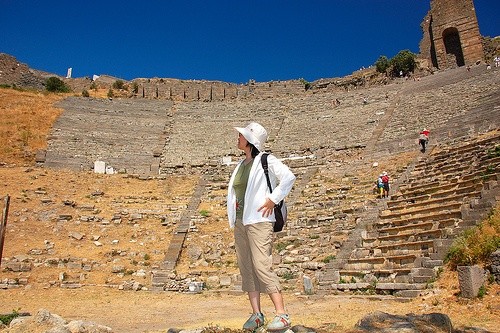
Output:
[243,312,264,330]
[267,313,290,331]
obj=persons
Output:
[377,171,389,199]
[419,128,430,153]
[227,121,295,331]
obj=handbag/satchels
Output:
[274,199,288,231]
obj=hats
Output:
[380,171,387,176]
[235,122,267,152]
[423,128,428,131]
